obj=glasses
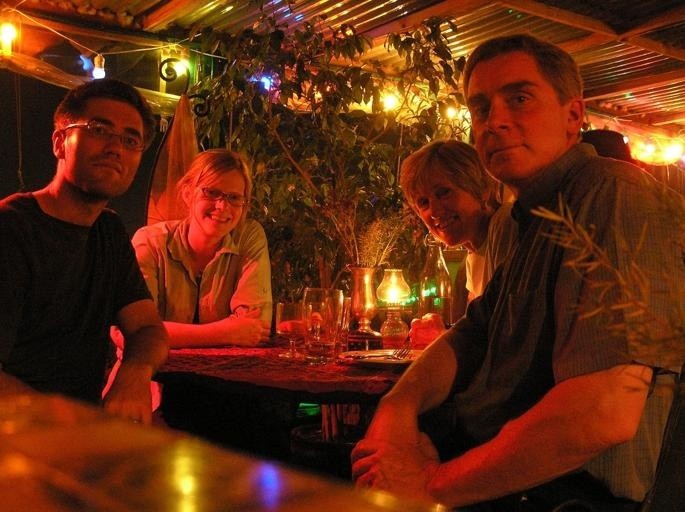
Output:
[197,186,245,208]
[64,119,146,153]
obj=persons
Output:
[0,78,172,429]
[131,150,273,350]
[350,33,685,510]
[398,137,519,313]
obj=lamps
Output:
[377,267,412,349]
[90,50,108,80]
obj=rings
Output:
[131,419,142,425]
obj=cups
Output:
[274,287,351,366]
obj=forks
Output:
[347,345,411,359]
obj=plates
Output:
[339,350,425,365]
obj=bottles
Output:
[419,240,455,336]
